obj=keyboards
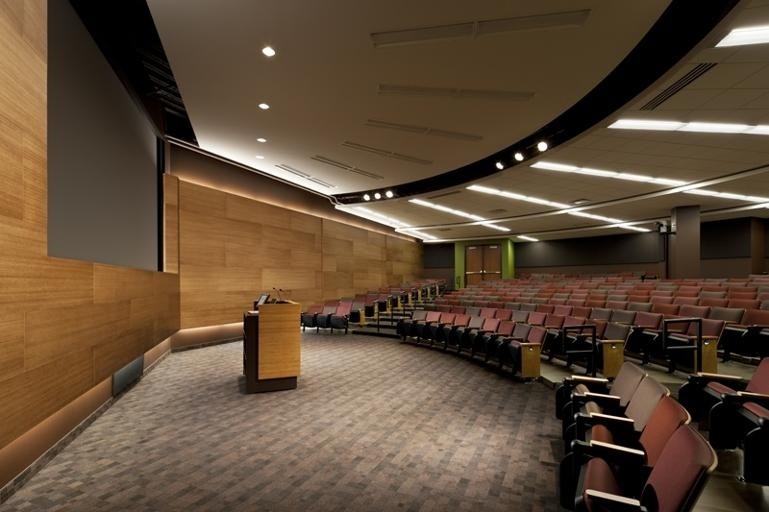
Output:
[248,311,259,314]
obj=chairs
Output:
[301,274,769,512]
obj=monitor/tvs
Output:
[255,294,270,307]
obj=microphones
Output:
[279,289,288,303]
[273,288,281,304]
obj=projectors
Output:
[659,221,668,232]
